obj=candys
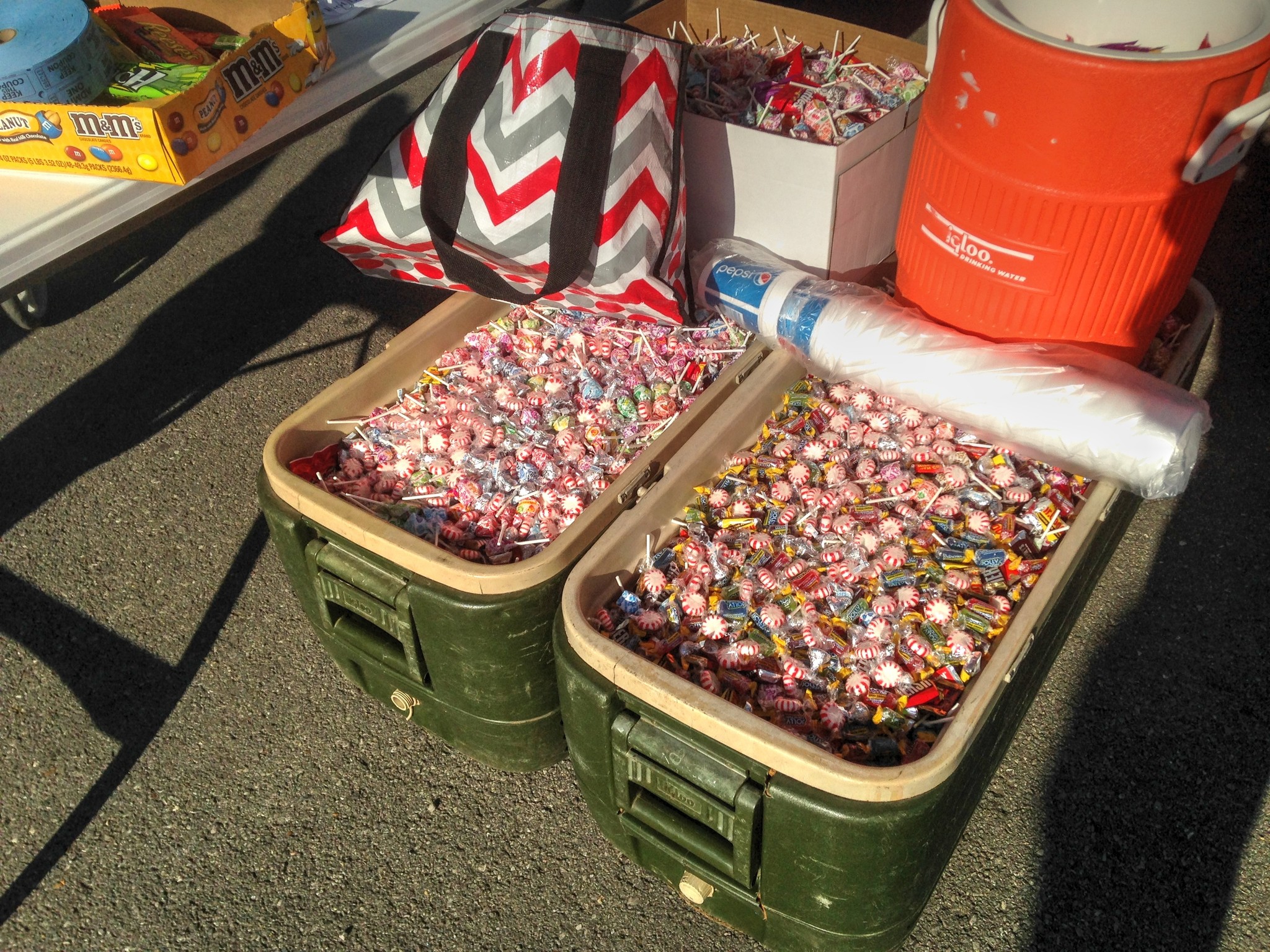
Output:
[592,277,1185,769]
[664,7,928,146]
[305,308,757,560]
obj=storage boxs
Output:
[551,260,1215,952]
[622,0,929,282]
[0,0,336,186]
[254,293,778,775]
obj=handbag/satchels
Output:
[318,10,691,327]
